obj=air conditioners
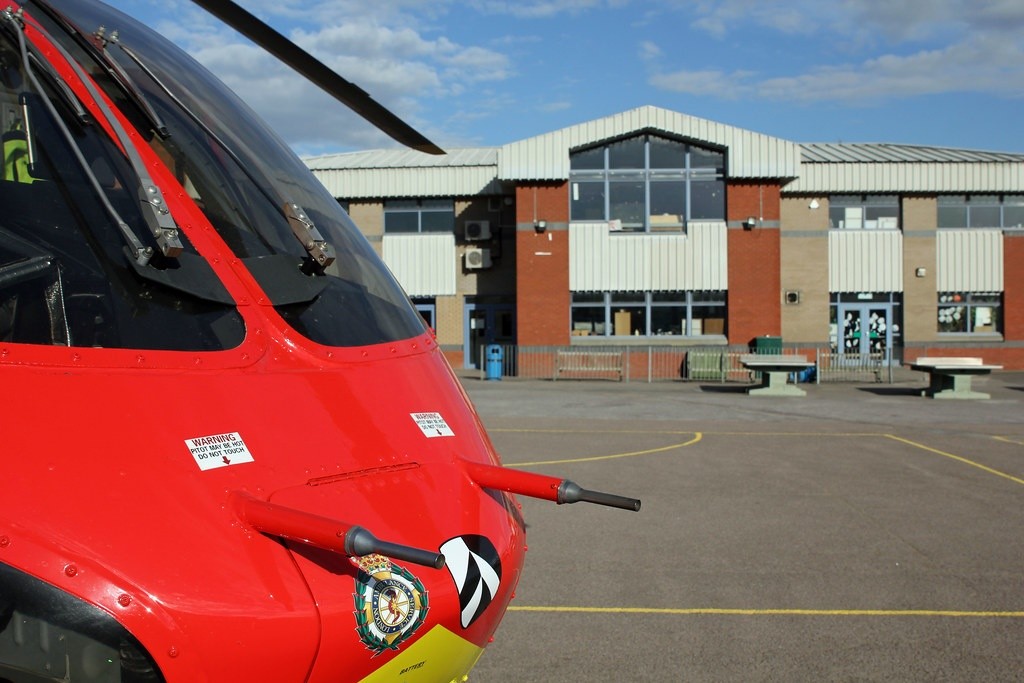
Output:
[465,248,493,269]
[464,221,491,240]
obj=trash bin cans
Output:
[484,343,504,380]
[747,336,782,379]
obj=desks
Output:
[738,354,815,397]
[903,357,1004,399]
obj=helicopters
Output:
[0,0,640,683]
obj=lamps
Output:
[748,216,756,228]
[916,268,926,277]
[538,220,546,231]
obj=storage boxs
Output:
[615,312,631,336]
[974,326,994,333]
[574,322,612,336]
[703,318,724,335]
[682,319,703,336]
[650,215,682,232]
